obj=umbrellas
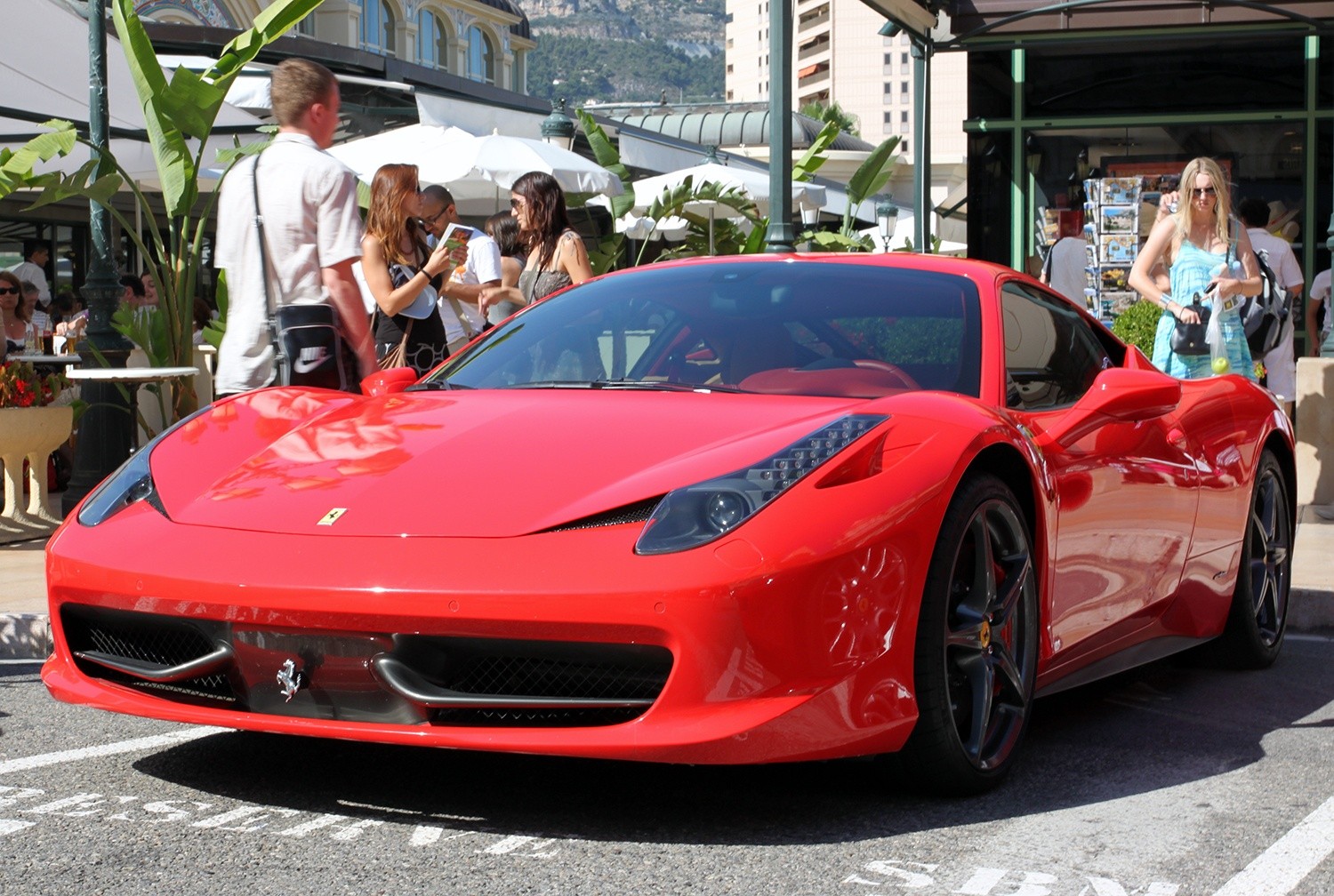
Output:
[614,162,827,256]
[326,125,625,215]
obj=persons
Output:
[360,164,451,380]
[215,58,378,402]
[419,185,502,355]
[0,271,88,365]
[1127,157,1262,385]
[478,171,594,311]
[11,237,52,310]
[119,266,166,349]
[1307,268,1332,357]
[1238,199,1304,418]
[483,210,529,332]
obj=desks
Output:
[64,367,200,458]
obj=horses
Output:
[277,659,300,702]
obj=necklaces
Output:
[7,317,16,327]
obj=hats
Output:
[389,265,437,320]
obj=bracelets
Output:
[1236,278,1243,295]
[1155,216,1160,224]
[421,269,433,281]
[1158,293,1172,310]
[0,324,6,327]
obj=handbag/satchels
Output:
[266,304,350,387]
[1169,292,1213,355]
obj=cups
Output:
[80,328,87,339]
[63,315,71,335]
[38,330,45,354]
[66,330,77,356]
[23,325,35,356]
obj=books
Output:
[436,223,474,270]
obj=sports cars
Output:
[41,252,1297,791]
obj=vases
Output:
[0,408,51,543]
[26,406,73,533]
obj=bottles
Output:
[43,314,53,356]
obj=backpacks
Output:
[1239,251,1294,355]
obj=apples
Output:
[1211,357,1229,374]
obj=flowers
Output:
[0,360,66,408]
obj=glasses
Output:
[1188,187,1218,198]
[413,185,421,195]
[0,287,20,296]
[508,198,525,211]
[419,205,450,226]
